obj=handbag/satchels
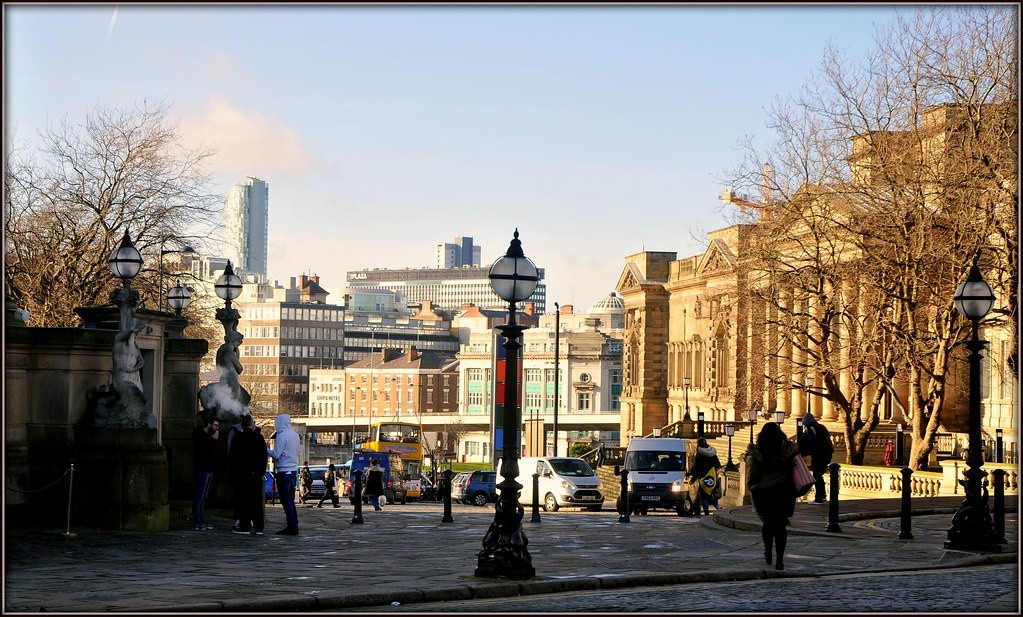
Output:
[792,454,817,496]
[378,495,387,506]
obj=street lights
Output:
[944,254,1007,553]
[368,326,375,437]
[353,386,362,456]
[409,383,422,425]
[211,257,246,418]
[391,378,400,437]
[477,226,539,581]
[94,227,156,430]
[158,247,196,312]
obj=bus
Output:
[347,449,411,506]
[351,421,423,502]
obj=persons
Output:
[364,459,385,510]
[226,407,268,535]
[798,413,834,504]
[316,464,341,508]
[216,329,252,405]
[190,416,224,530]
[747,422,798,570]
[884,437,895,466]
[689,437,721,515]
[638,452,678,470]
[336,476,346,498]
[300,461,313,504]
[400,434,411,443]
[267,413,301,535]
[111,317,146,393]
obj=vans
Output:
[615,436,699,516]
[494,456,606,512]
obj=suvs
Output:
[450,470,475,506]
[461,470,498,507]
[297,468,340,504]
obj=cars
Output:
[263,471,279,504]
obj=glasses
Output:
[212,424,220,427]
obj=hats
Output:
[802,413,814,425]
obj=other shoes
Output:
[823,498,827,502]
[193,523,214,531]
[233,520,240,528]
[317,504,323,508]
[334,504,341,507]
[808,500,824,505]
[715,505,722,509]
[232,528,250,534]
[764,552,772,564]
[299,496,306,504]
[255,529,264,535]
[775,560,784,570]
[277,525,298,535]
